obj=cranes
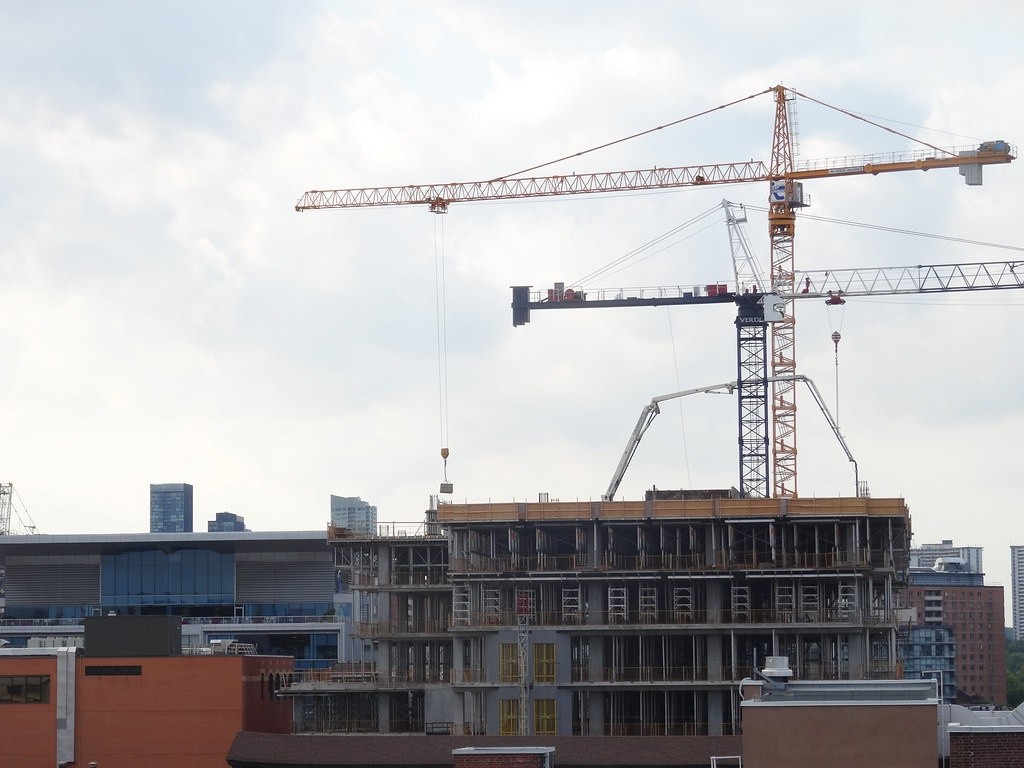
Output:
[0,480,39,536]
[508,200,1024,496]
[295,79,1020,500]
